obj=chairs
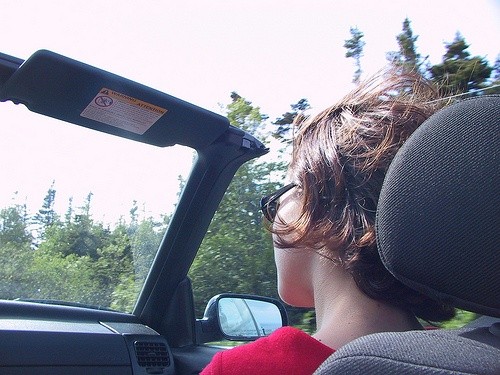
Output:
[311,93,500,375]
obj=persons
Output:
[198,62,458,375]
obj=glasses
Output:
[260,182,302,223]
